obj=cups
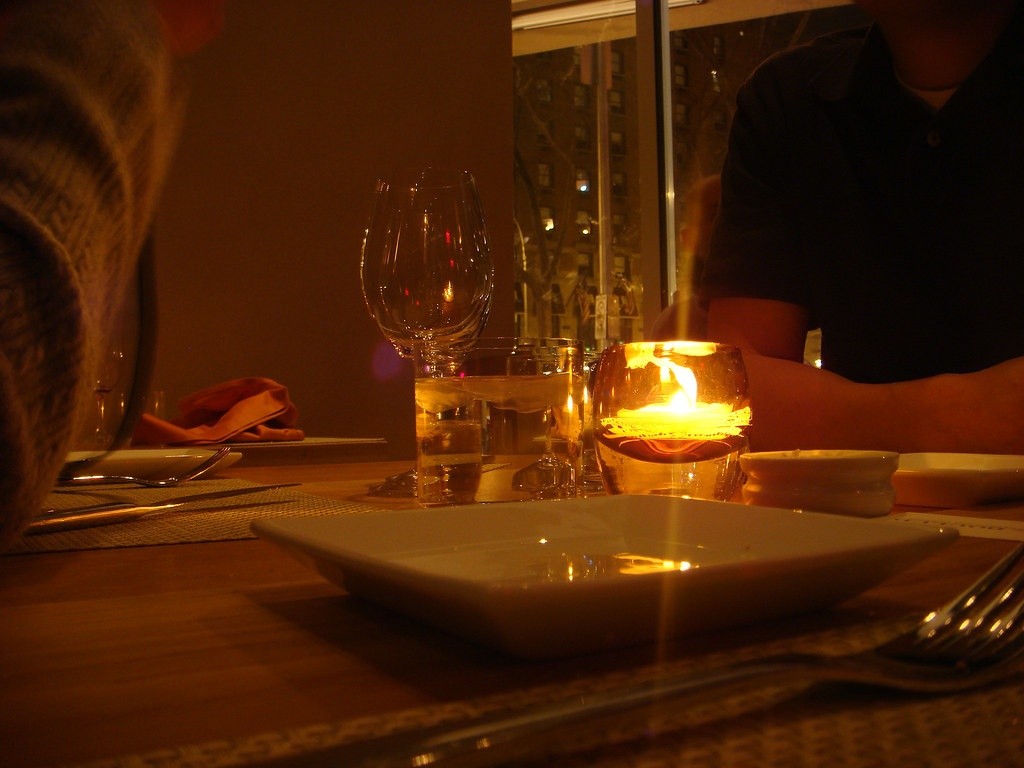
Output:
[594,342,753,500]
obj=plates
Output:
[63,449,243,481]
[891,451,1024,509]
[247,493,961,669]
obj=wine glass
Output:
[359,163,495,507]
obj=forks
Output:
[58,446,231,488]
[254,543,1024,768]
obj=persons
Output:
[648,0,1024,453]
[0,0,227,555]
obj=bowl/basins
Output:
[738,450,901,518]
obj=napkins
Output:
[135,377,302,450]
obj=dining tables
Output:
[0,435,1024,768]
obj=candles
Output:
[592,340,752,506]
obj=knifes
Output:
[22,483,301,535]
